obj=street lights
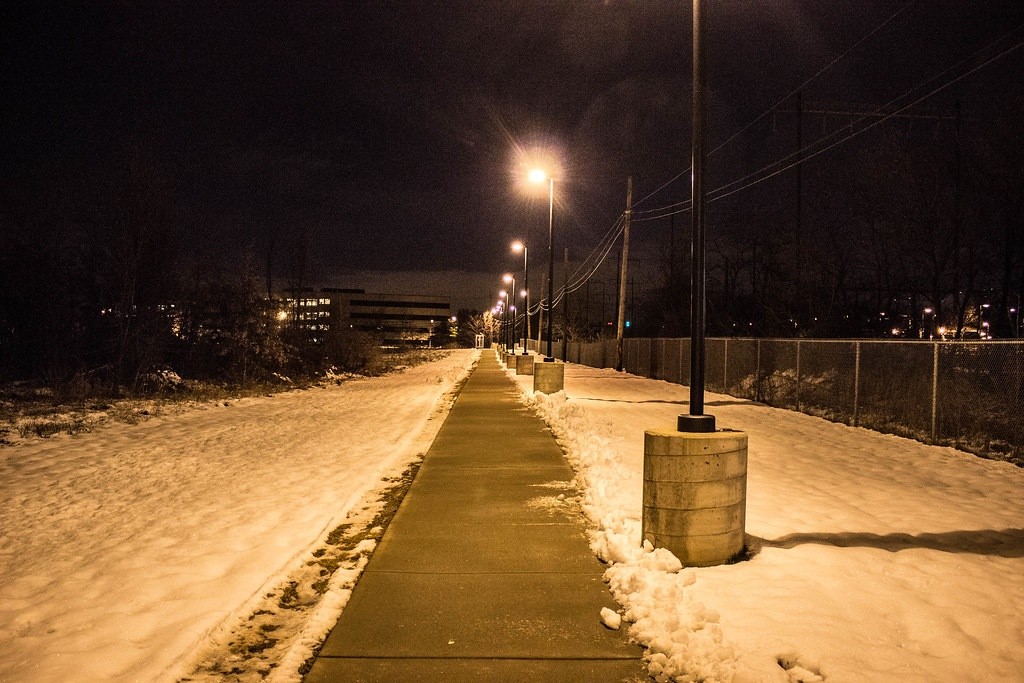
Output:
[530,167,565,394]
[495,306,503,352]
[497,300,506,355]
[504,275,518,369]
[501,291,510,363]
[498,291,509,359]
[512,240,534,375]
